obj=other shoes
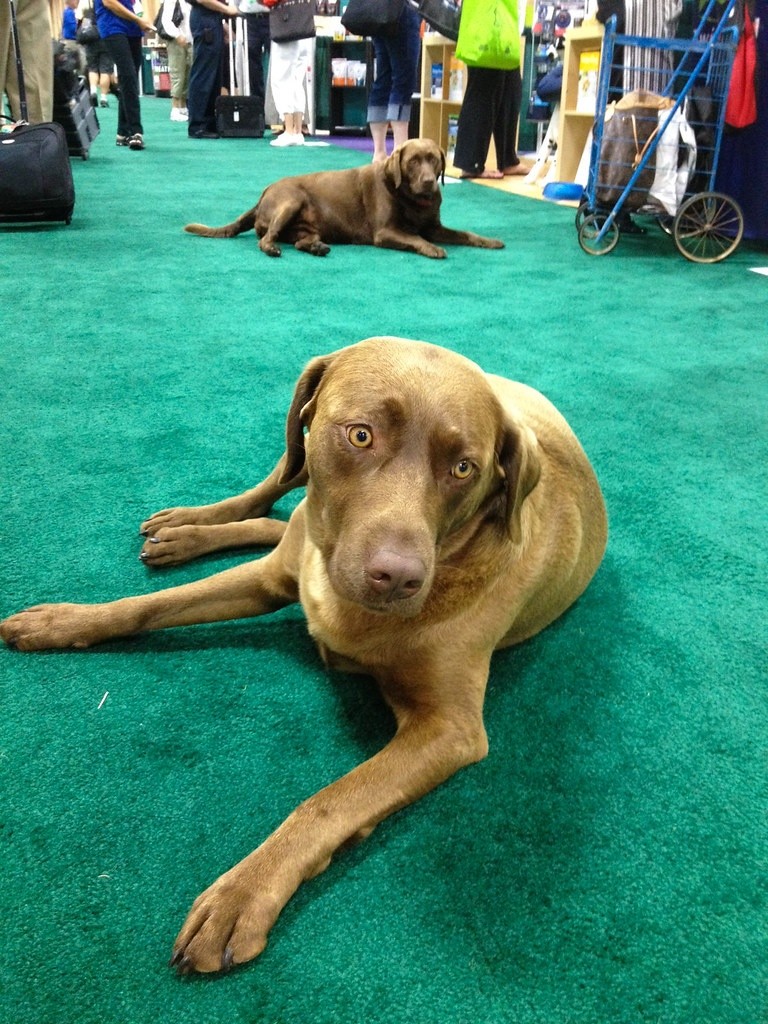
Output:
[101,100,109,108]
[587,210,645,235]
[270,131,305,147]
[90,93,99,108]
[192,128,220,138]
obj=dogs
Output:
[184,138,505,259]
[0,335,608,979]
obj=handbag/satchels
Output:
[724,0,758,128]
[597,108,657,207]
[269,0,316,44]
[153,0,183,40]
[340,0,407,36]
[453,0,519,70]
[76,17,99,42]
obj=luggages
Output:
[0,113,74,225]
[214,14,266,138]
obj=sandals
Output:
[115,136,128,145]
[129,134,144,147]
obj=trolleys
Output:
[576,0,744,266]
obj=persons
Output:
[80,0,115,108]
[262,0,313,146]
[366,0,424,163]
[184,0,237,139]
[454,0,531,179]
[94,0,157,146]
[62,0,79,40]
[0,0,53,123]
[161,0,194,121]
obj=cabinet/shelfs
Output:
[317,33,529,178]
[556,26,605,185]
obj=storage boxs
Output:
[431,62,443,100]
[446,114,460,161]
[448,53,467,103]
[53,74,101,150]
[576,47,602,114]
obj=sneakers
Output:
[170,108,190,122]
